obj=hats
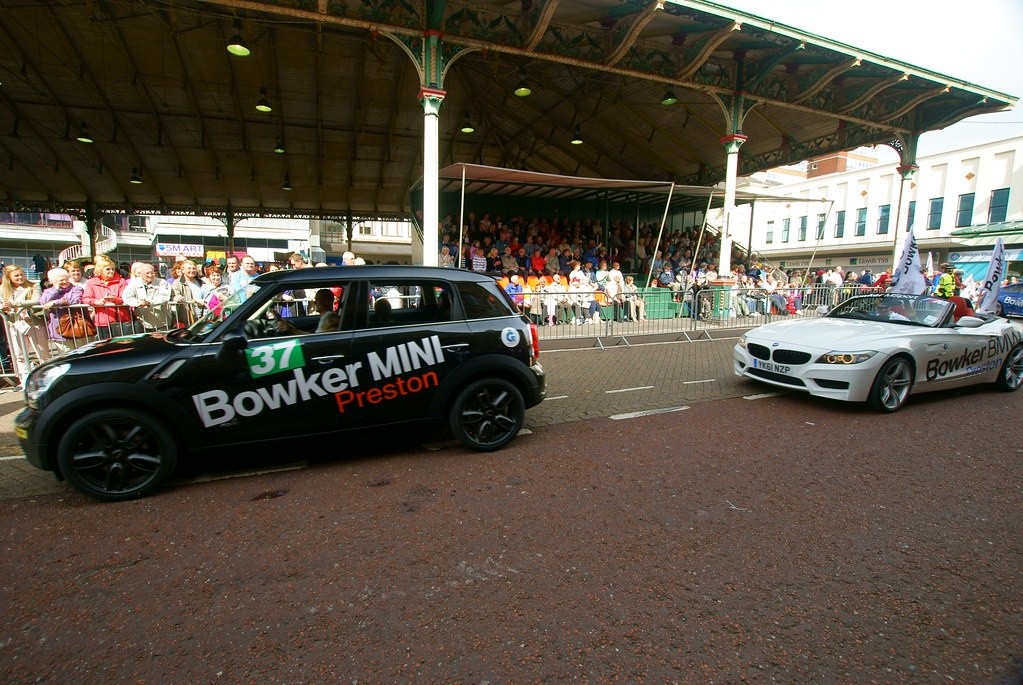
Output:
[941,263,952,269]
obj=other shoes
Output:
[738,307,804,318]
[531,316,647,327]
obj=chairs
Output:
[370,300,394,328]
[937,297,973,321]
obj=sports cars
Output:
[733,292,1023,414]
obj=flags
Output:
[927,253,933,276]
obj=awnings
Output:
[950,260,1008,284]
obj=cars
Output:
[11,264,546,502]
[979,283,1023,316]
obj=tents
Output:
[408,161,834,319]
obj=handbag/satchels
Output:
[56,311,97,339]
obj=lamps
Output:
[273,144,284,153]
[129,176,142,184]
[515,81,531,96]
[282,183,291,191]
[77,133,93,143]
[255,98,271,112]
[225,32,250,56]
[460,122,474,133]
[570,133,583,145]
[661,91,677,105]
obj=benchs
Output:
[561,256,680,322]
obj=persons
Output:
[609,218,804,320]
[779,262,1017,308]
[416,209,648,327]
[0,251,402,390]
[375,299,394,323]
[276,289,340,335]
